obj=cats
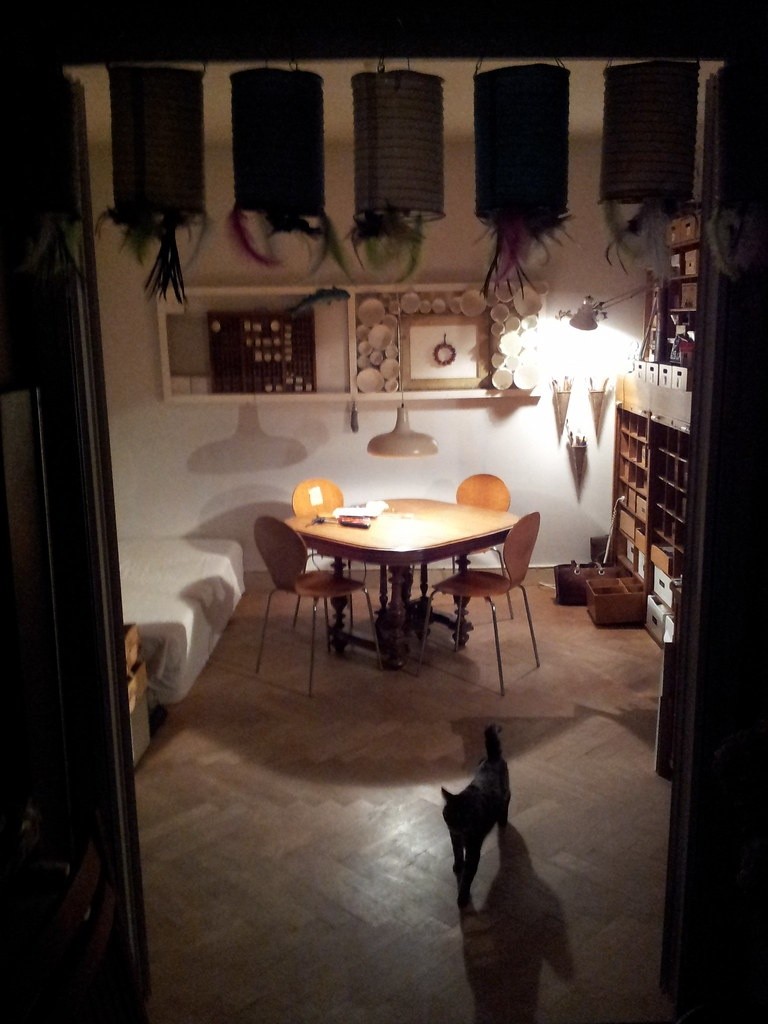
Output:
[440,722,512,909]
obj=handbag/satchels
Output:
[555,559,618,606]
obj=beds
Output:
[117,537,246,708]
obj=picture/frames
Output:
[399,313,490,391]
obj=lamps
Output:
[367,286,440,458]
[569,285,652,331]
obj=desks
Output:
[284,498,521,670]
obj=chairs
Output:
[253,516,384,698]
[291,477,354,630]
[452,473,514,621]
[415,511,541,698]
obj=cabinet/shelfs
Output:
[611,373,692,649]
[644,201,702,370]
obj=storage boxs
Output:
[637,495,647,521]
[654,565,675,609]
[619,509,635,539]
[665,218,680,248]
[671,366,694,392]
[128,659,150,713]
[681,282,699,308]
[123,623,139,675]
[684,249,700,275]
[638,550,646,579]
[635,360,646,381]
[646,595,675,642]
[681,209,702,247]
[659,363,672,389]
[626,539,634,563]
[663,615,674,643]
[629,487,636,511]
[650,543,673,576]
[645,362,659,385]
[585,576,646,626]
[635,527,646,554]
